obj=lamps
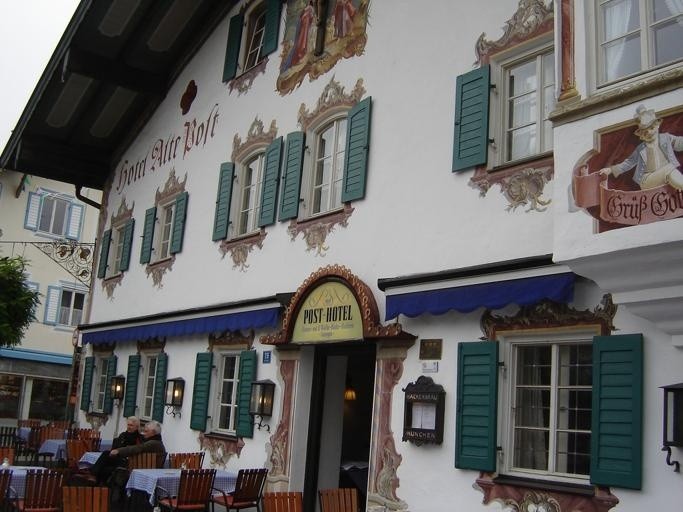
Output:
[249,379,276,432]
[345,389,357,405]
[657,382,683,472]
[164,377,184,419]
[109,374,125,408]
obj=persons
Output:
[284,0,357,70]
[79,416,167,482]
[598,109,683,189]
